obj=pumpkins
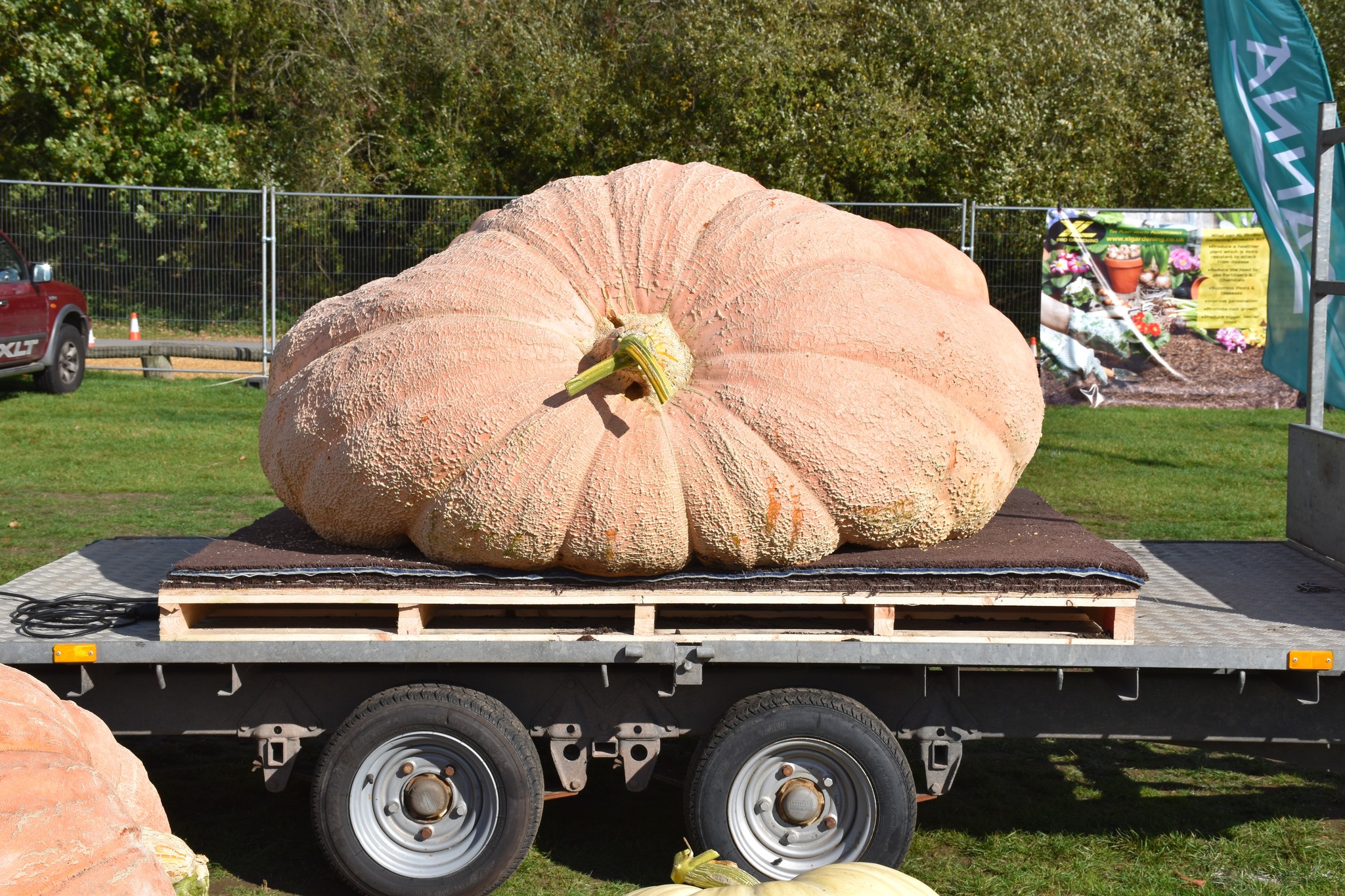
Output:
[257,158,1045,579]
[613,849,941,896]
[0,664,210,896]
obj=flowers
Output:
[1041,249,1093,294]
[1107,243,1143,261]
[1166,248,1203,288]
[1215,327,1267,352]
[1118,311,1171,364]
[1059,275,1101,307]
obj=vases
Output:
[1129,341,1154,369]
[1050,285,1067,300]
[1067,298,1093,313]
[1104,254,1144,294]
[1172,280,1192,300]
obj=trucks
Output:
[0,99,1345,896]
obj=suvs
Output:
[0,228,93,394]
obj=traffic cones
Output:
[130,312,140,340]
[88,328,95,349]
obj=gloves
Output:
[1040,324,1109,385]
[1068,306,1135,359]
